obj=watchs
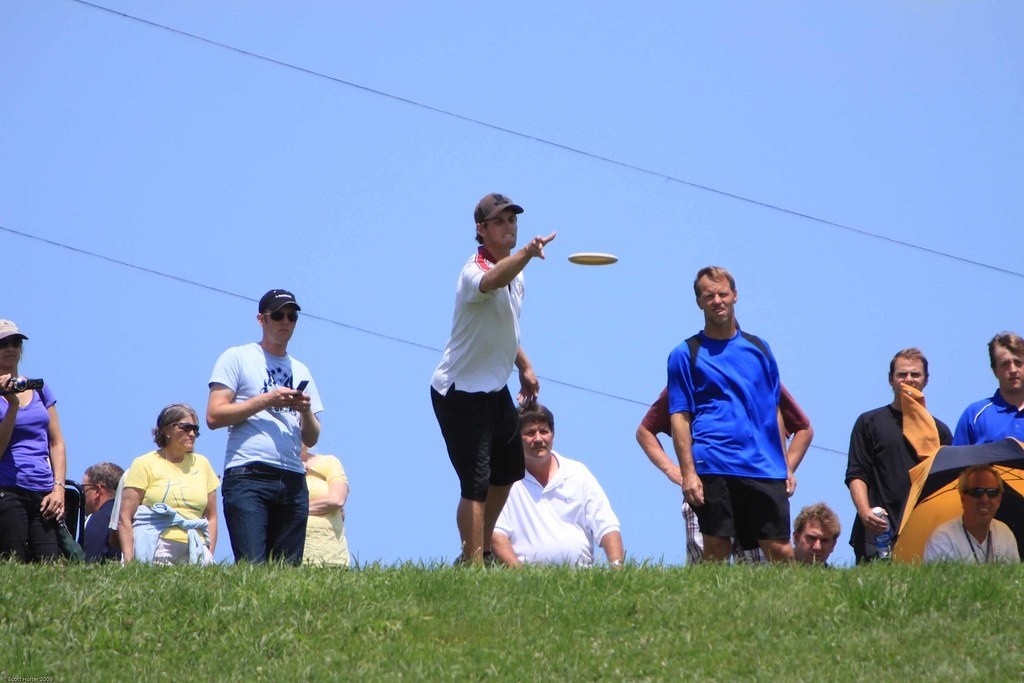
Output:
[609,559,624,568]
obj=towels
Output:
[900,382,940,459]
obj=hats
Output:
[475,193,524,223]
[259,289,301,313]
[0,320,29,339]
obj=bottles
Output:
[873,507,895,559]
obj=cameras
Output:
[6,377,44,393]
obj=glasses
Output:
[0,338,23,349]
[82,484,105,489]
[172,423,200,433]
[263,312,298,322]
[963,488,1000,499]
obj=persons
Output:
[430,191,557,573]
[951,331,1024,446]
[489,401,626,567]
[844,347,954,566]
[764,501,841,565]
[923,466,1022,566]
[635,265,814,566]
[82,462,125,564]
[206,289,325,570]
[113,402,222,569]
[301,442,351,568]
[0,319,67,564]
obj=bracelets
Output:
[54,481,66,491]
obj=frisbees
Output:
[567,252,619,266]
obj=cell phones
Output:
[295,381,309,396]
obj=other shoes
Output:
[453,550,494,566]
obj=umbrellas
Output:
[57,517,87,562]
